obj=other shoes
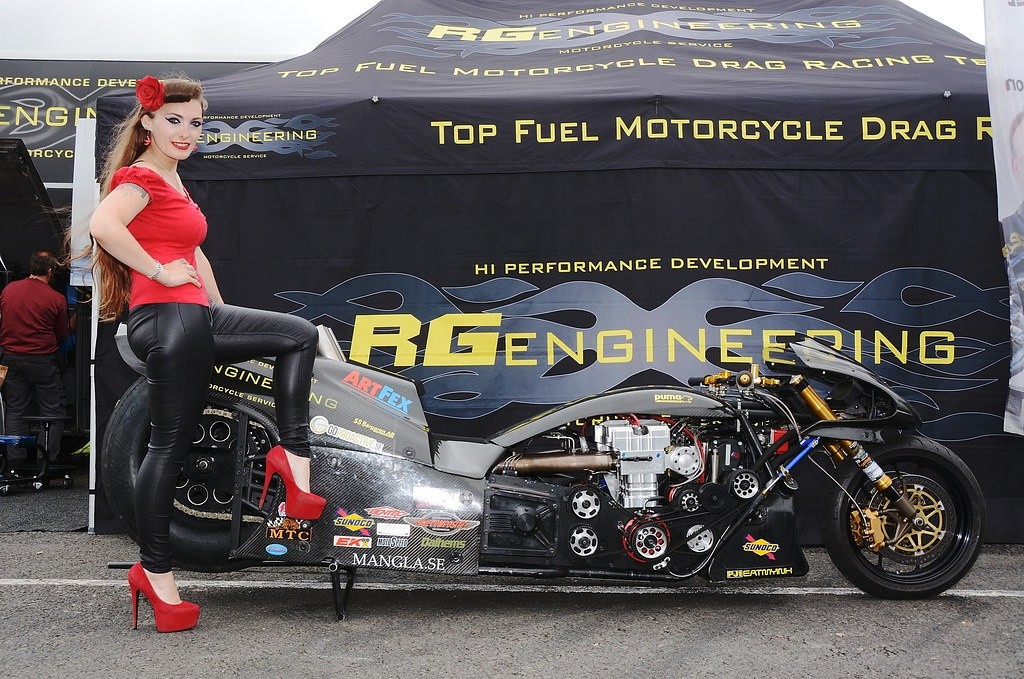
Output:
[33,472,48,491]
[5,471,27,485]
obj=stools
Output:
[0,414,73,497]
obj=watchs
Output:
[147,259,163,280]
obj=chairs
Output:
[315,324,427,398]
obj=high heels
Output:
[128,562,200,633]
[259,445,326,520]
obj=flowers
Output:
[134,75,166,112]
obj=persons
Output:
[64,67,325,633]
[0,247,78,497]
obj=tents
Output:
[88,0,1023,546]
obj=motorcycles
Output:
[98,324,990,621]
